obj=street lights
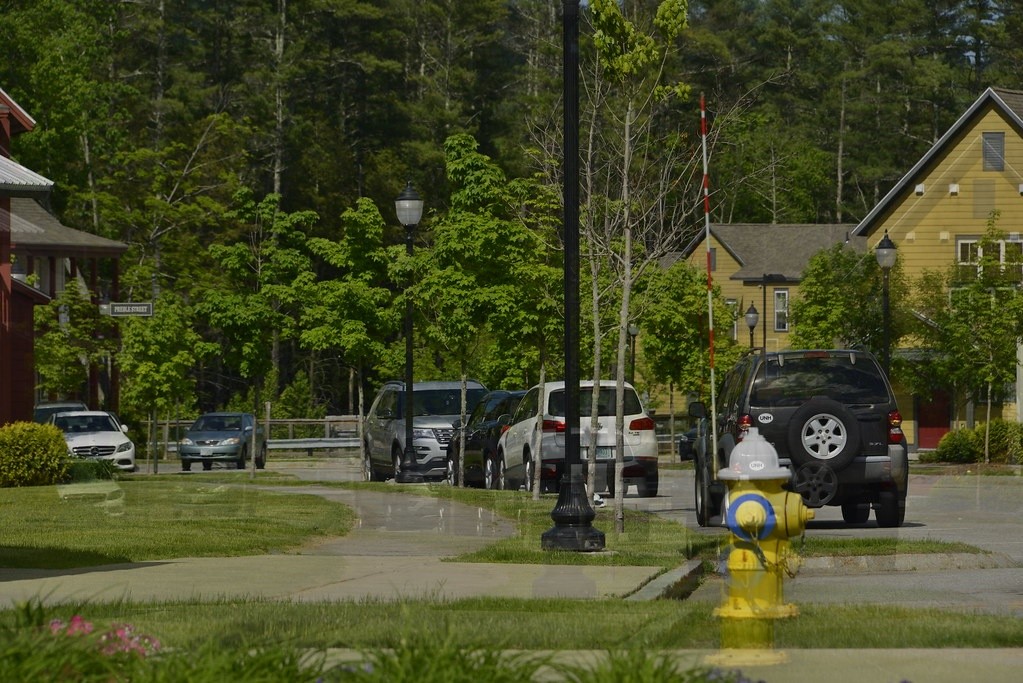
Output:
[628,321,639,385]
[744,299,759,348]
[392,182,426,483]
[876,228,898,380]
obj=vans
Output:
[496,380,659,501]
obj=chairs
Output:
[58,419,68,432]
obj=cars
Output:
[179,411,267,470]
[46,411,136,472]
[53,482,126,521]
[679,418,710,461]
[445,389,530,489]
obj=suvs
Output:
[362,379,490,483]
[689,349,908,528]
[31,402,93,430]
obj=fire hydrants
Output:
[713,429,815,619]
